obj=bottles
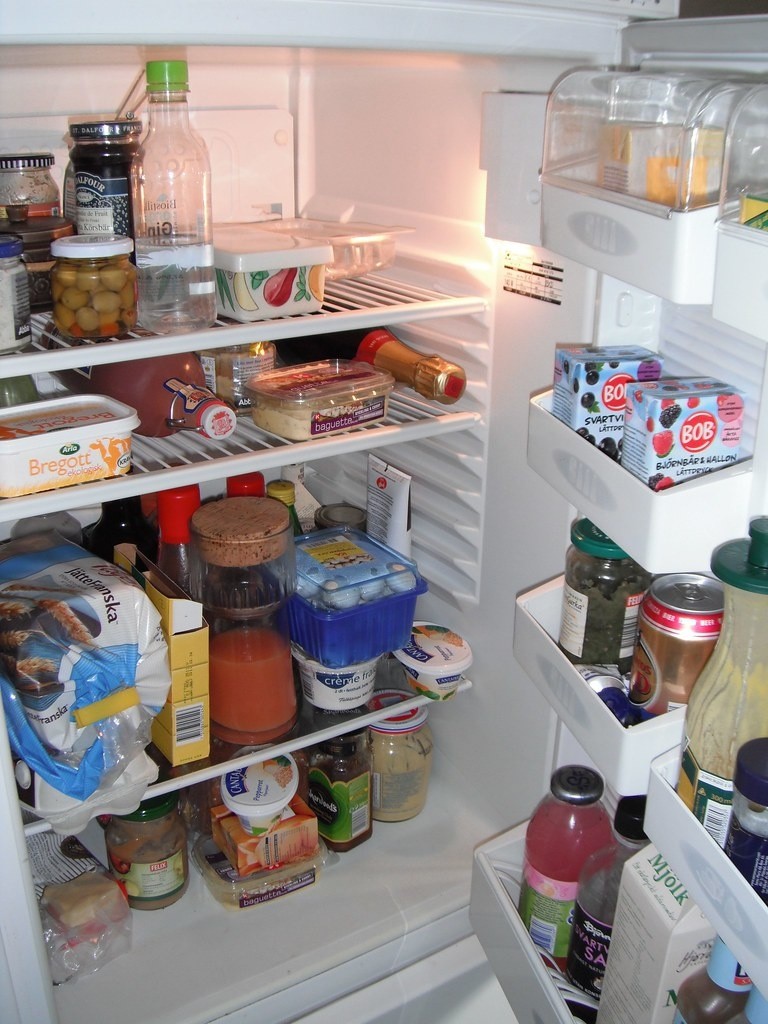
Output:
[129,59,220,336]
[11,464,306,745]
[673,933,752,1024]
[566,796,651,1000]
[271,298,467,406]
[40,319,237,440]
[518,764,616,973]
[726,983,768,1024]
[677,517,768,849]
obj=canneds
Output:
[0,217,73,313]
[105,775,189,909]
[0,153,60,217]
[628,573,724,723]
[308,703,372,851]
[51,234,137,337]
[314,503,366,534]
[362,689,433,822]
[0,235,32,354]
[68,120,143,266]
[559,519,652,674]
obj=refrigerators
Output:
[0,0,768,1024]
